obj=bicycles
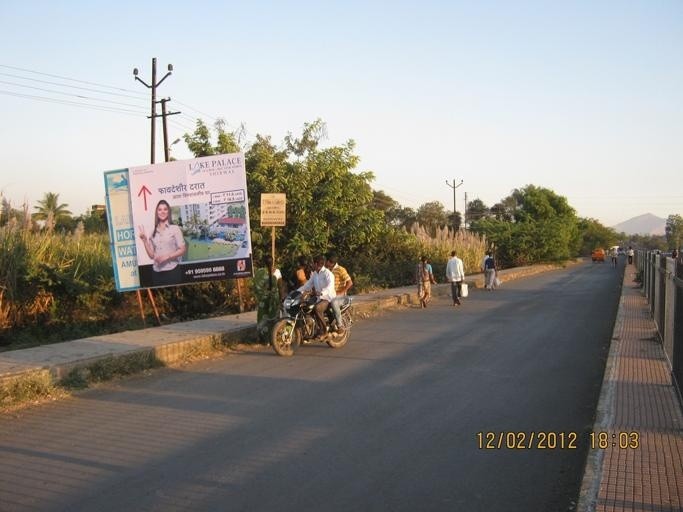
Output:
[610,254,617,271]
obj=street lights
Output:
[444,178,464,214]
[132,58,176,165]
[165,137,181,163]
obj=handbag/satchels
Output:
[460,284,469,297]
[493,278,500,288]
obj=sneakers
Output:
[337,327,345,336]
[453,298,460,306]
[320,332,329,342]
[420,299,427,309]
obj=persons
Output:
[610,248,618,269]
[416,256,435,311]
[253,257,284,346]
[672,249,678,258]
[137,200,186,286]
[480,252,500,291]
[446,251,465,307]
[626,246,634,265]
[289,256,352,341]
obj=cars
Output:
[590,247,605,264]
[617,250,626,256]
[647,249,661,254]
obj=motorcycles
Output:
[269,284,355,360]
